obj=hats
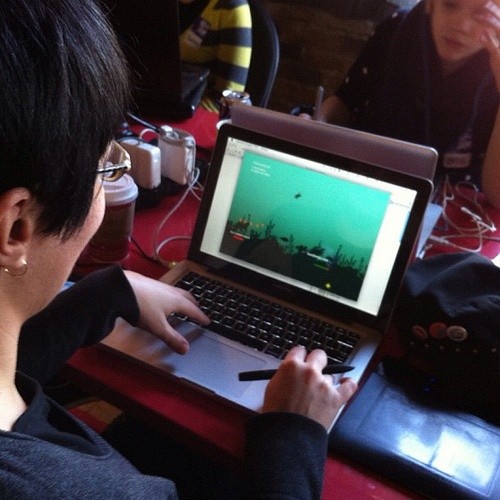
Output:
[391,251,500,380]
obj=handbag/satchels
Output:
[134,57,211,119]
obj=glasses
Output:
[94,139,131,182]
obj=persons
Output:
[0,0,357,500]
[178,0,253,117]
[312,0,500,207]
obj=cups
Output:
[86,172,138,263]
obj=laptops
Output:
[94,122,436,440]
[225,103,445,263]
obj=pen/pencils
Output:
[313,86,324,120]
[238,366,355,382]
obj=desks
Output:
[66,108,500,500]
